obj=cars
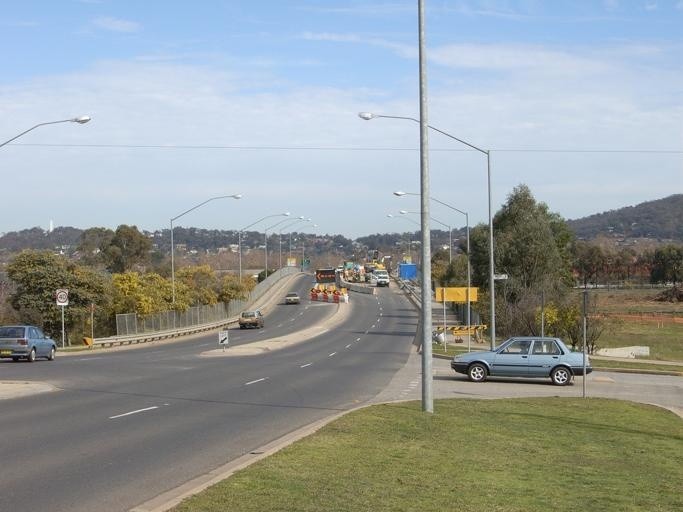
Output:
[0,325,57,362]
[452,337,592,384]
[286,293,300,304]
[239,310,264,329]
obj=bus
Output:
[343,261,365,282]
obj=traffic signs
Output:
[55,289,69,306]
[219,330,229,344]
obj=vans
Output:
[364,262,389,287]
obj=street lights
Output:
[238,212,290,298]
[387,190,471,354]
[265,217,318,277]
[361,113,497,351]
[0,117,91,149]
[170,195,242,304]
